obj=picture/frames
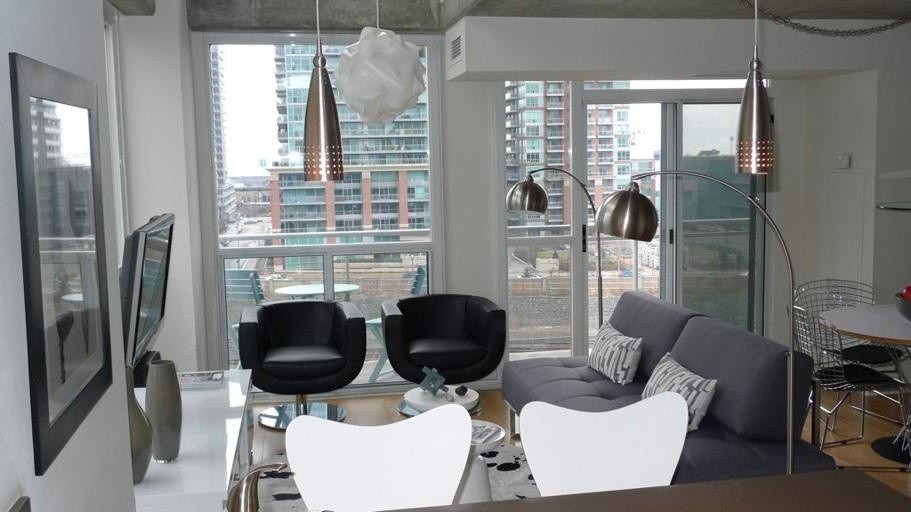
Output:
[8,51,113,474]
[144,360,183,463]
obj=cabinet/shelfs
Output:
[134,369,255,512]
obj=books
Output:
[472,422,502,444]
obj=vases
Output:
[125,366,153,484]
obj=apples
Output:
[903,287,911,300]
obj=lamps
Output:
[336,0,426,125]
[595,171,794,475]
[734,0,775,177]
[505,168,602,327]
[303,0,345,182]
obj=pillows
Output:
[588,319,643,385]
[640,351,718,431]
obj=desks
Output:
[819,305,911,464]
[384,467,911,512]
[275,284,359,299]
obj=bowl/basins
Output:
[894,291,911,323]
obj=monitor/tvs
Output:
[120,212,175,367]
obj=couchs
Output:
[502,290,835,485]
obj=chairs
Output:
[224,267,265,369]
[786,303,911,453]
[285,405,472,511]
[237,299,366,429]
[520,391,689,497]
[366,262,427,382]
[381,294,506,415]
[794,278,911,432]
